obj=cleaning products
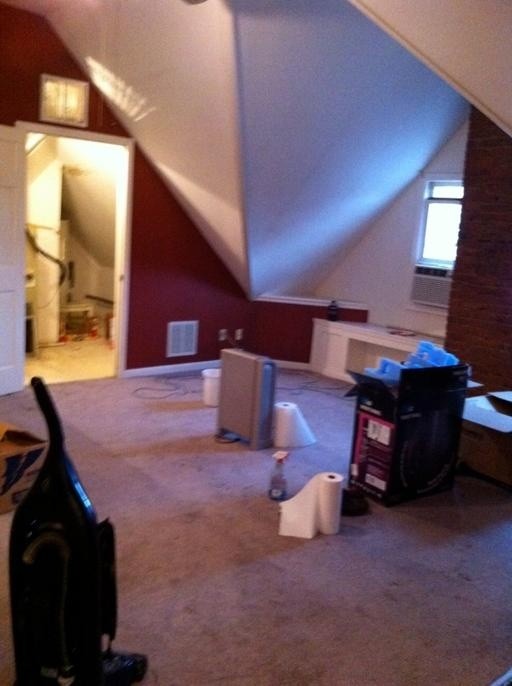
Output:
[267,448,289,501]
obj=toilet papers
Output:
[274,402,315,449]
[279,471,344,540]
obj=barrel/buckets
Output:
[202,368,220,406]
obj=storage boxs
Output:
[343,361,512,510]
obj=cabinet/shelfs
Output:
[307,316,445,383]
